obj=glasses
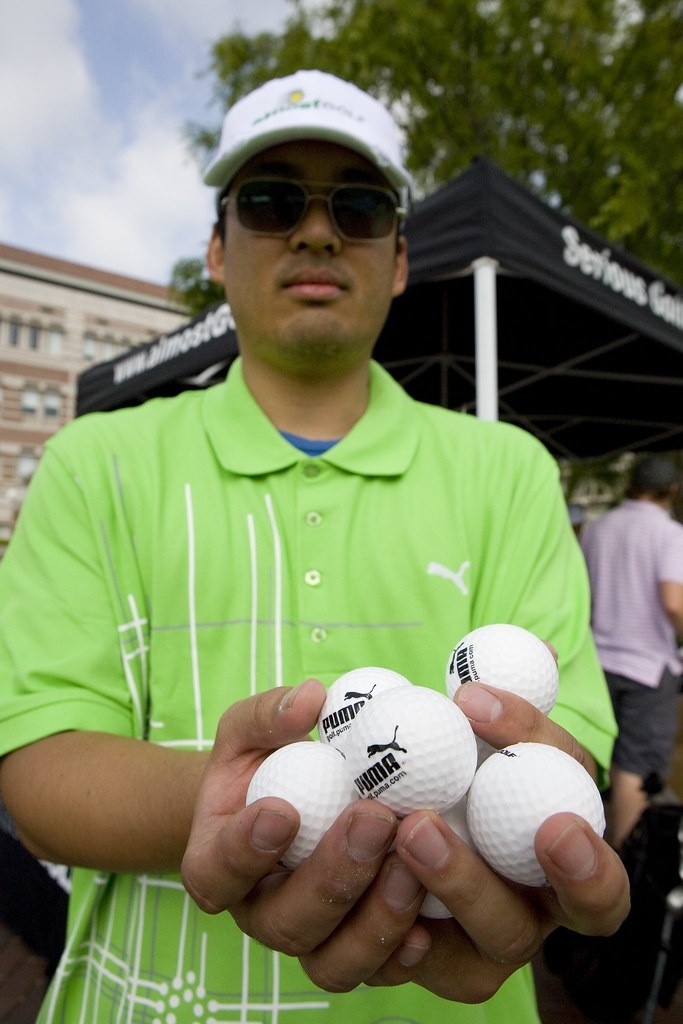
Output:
[221,178,408,243]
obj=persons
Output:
[0,63,628,1024]
[581,451,682,846]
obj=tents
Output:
[72,154,682,428]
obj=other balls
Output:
[316,664,417,758]
[384,790,486,922]
[466,742,606,892]
[443,623,561,727]
[243,737,361,875]
[345,685,477,821]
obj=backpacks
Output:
[541,805,683,1024]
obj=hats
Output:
[630,457,677,486]
[204,70,413,229]
